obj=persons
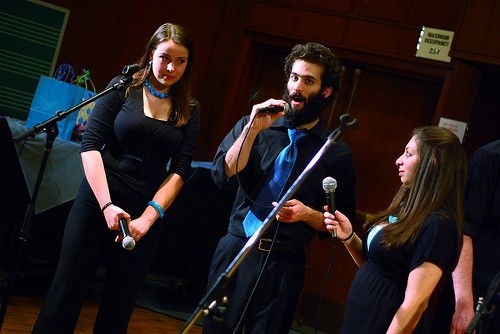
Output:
[32,22,200,334]
[450,139,500,334]
[323,126,468,334]
[204,41,357,334]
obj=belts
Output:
[256,238,305,254]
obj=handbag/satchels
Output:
[25,63,99,144]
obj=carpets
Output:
[134,302,325,334]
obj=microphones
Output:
[259,102,290,114]
[118,217,136,250]
[322,177,338,237]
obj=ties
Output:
[242,127,311,239]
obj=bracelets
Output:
[148,201,165,218]
[340,229,354,242]
[102,202,113,212]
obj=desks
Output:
[0,115,84,287]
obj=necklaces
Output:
[145,79,171,99]
[389,213,399,223]
